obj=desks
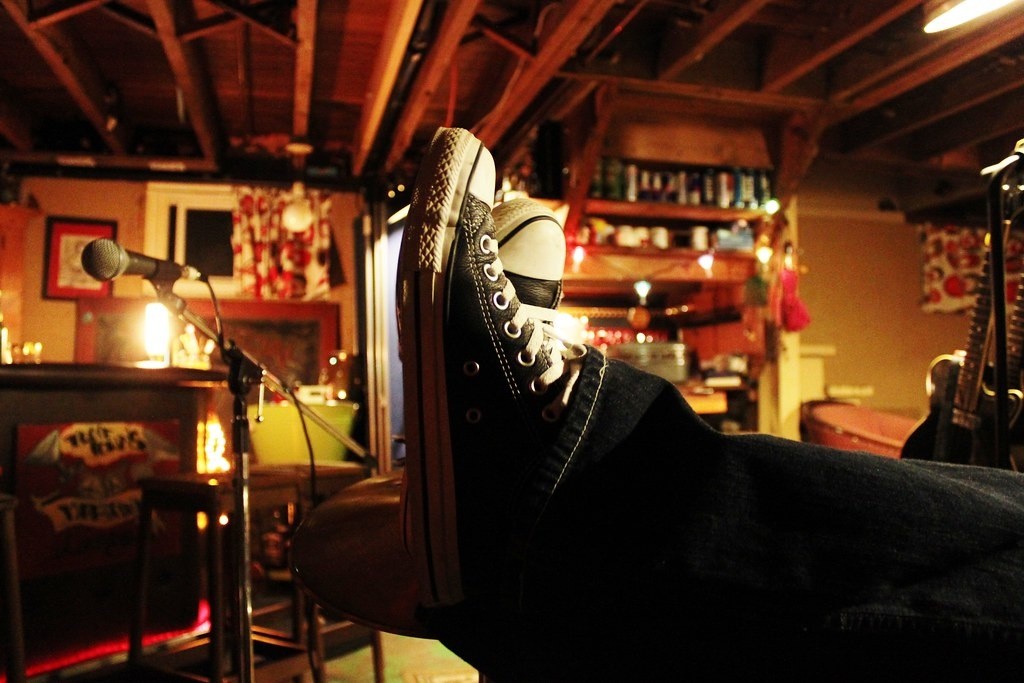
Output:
[290,471,564,683]
[0,361,232,683]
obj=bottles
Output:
[591,160,771,211]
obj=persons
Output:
[396,125,1024,683]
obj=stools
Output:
[0,462,385,683]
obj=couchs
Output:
[802,398,921,463]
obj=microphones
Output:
[81,238,208,282]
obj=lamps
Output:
[921,1,1023,33]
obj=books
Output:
[594,165,771,209]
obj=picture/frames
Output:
[41,214,117,302]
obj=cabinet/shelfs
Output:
[557,157,775,283]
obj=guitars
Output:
[900,149,1024,472]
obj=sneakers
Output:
[395,126,588,613]
[490,199,567,326]
